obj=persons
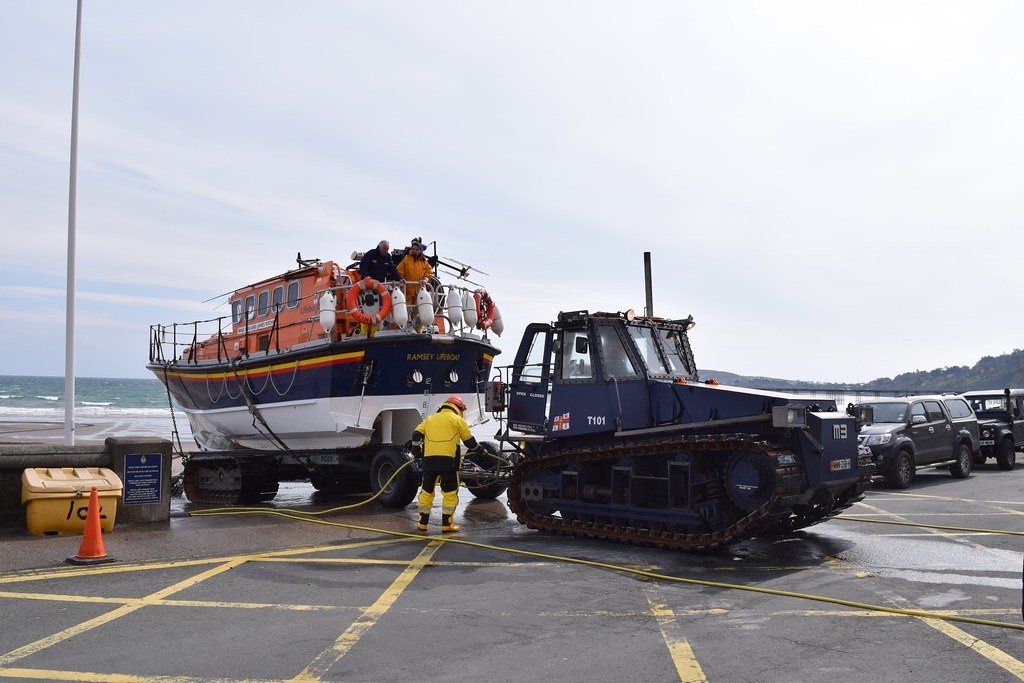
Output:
[412,396,488,531]
[397,242,433,333]
[605,333,630,375]
[361,240,407,329]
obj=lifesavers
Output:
[347,276,391,325]
[474,288,497,331]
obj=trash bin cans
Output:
[21,467,124,538]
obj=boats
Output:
[146,240,502,465]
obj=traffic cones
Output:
[66,486,117,565]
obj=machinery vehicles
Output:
[492,251,878,553]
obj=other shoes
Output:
[443,524,460,531]
[416,521,429,530]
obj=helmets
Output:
[447,395,468,411]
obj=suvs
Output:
[958,387,1024,472]
[848,392,982,489]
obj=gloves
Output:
[413,451,422,462]
[479,447,488,457]
